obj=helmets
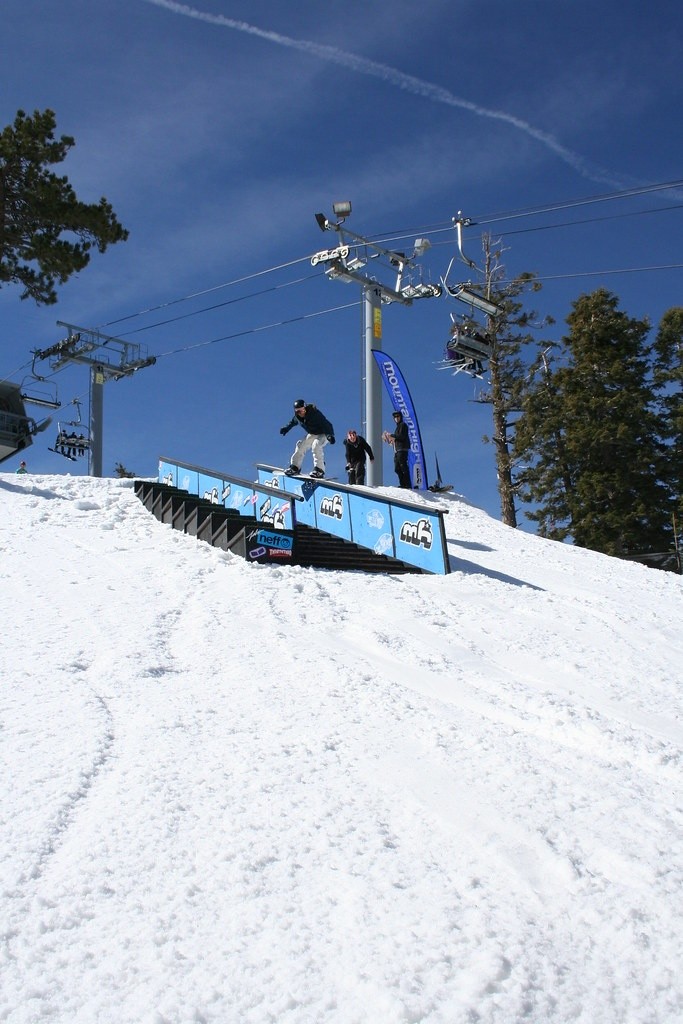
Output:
[294,400,305,407]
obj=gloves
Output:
[280,426,288,436]
[326,436,334,445]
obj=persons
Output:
[390,411,412,488]
[15,462,27,474]
[79,434,84,456]
[53,430,67,454]
[280,399,336,477]
[447,324,488,378]
[343,431,375,485]
[67,432,77,456]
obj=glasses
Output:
[296,408,304,414]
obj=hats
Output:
[392,411,402,417]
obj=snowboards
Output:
[273,470,338,481]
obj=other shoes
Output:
[309,467,323,479]
[284,466,299,476]
